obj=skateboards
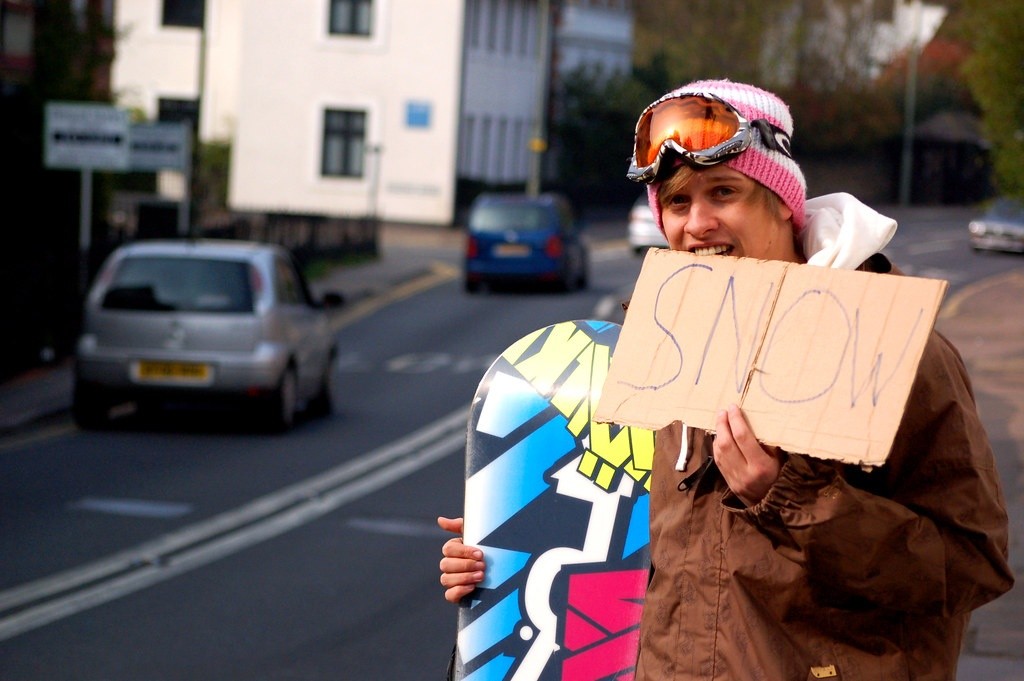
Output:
[447,317,659,680]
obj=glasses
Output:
[626,93,792,181]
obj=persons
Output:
[437,80,1016,681]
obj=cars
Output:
[71,237,345,436]
[461,186,590,293]
[967,192,1024,257]
[627,189,671,254]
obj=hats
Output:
[648,79,806,244]
